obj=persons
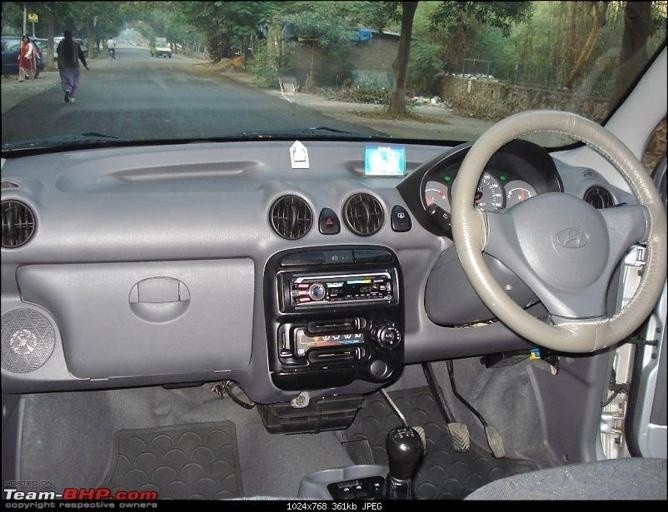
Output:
[56,31,91,102]
[17,34,36,82]
[107,35,116,59]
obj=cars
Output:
[1,35,88,80]
[150,41,172,58]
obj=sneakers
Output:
[64,90,71,104]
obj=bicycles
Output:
[109,50,114,61]
